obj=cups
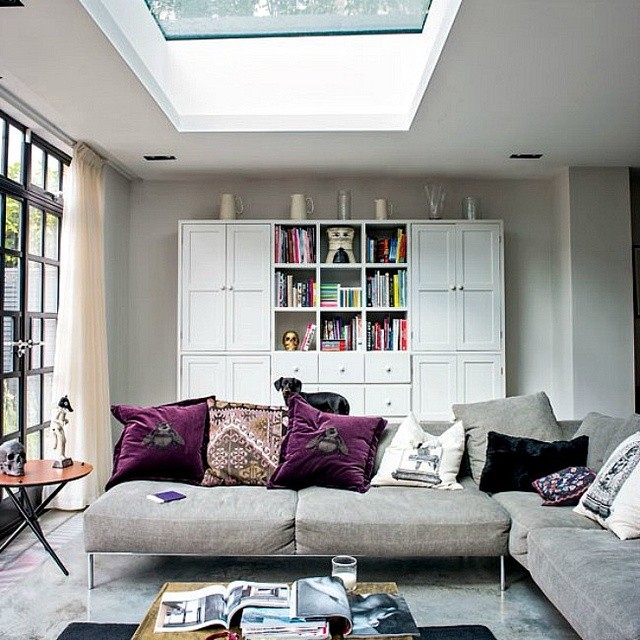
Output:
[338,190,351,219]
[219,194,244,219]
[331,555,357,593]
[374,199,392,219]
[288,193,313,219]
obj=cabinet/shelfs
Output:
[410,352,507,422]
[271,219,410,352]
[174,355,271,407]
[177,219,274,353]
[272,350,410,425]
[409,219,505,352]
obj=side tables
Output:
[1,460,93,576]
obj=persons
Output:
[1,442,27,476]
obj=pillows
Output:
[169,395,215,473]
[452,392,567,484]
[104,401,207,492]
[266,393,387,493]
[573,432,640,540]
[571,410,622,475]
[533,468,597,506]
[369,411,466,490]
[203,398,288,485]
[480,432,589,493]
[603,414,640,467]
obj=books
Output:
[301,323,318,353]
[364,265,409,312]
[364,309,411,353]
[274,225,319,268]
[155,580,289,632]
[145,483,183,509]
[238,607,331,640]
[272,268,318,310]
[318,311,364,353]
[318,268,363,311]
[294,576,419,635]
[364,222,409,267]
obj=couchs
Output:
[487,421,639,640]
[83,422,512,592]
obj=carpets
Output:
[56,623,497,640]
